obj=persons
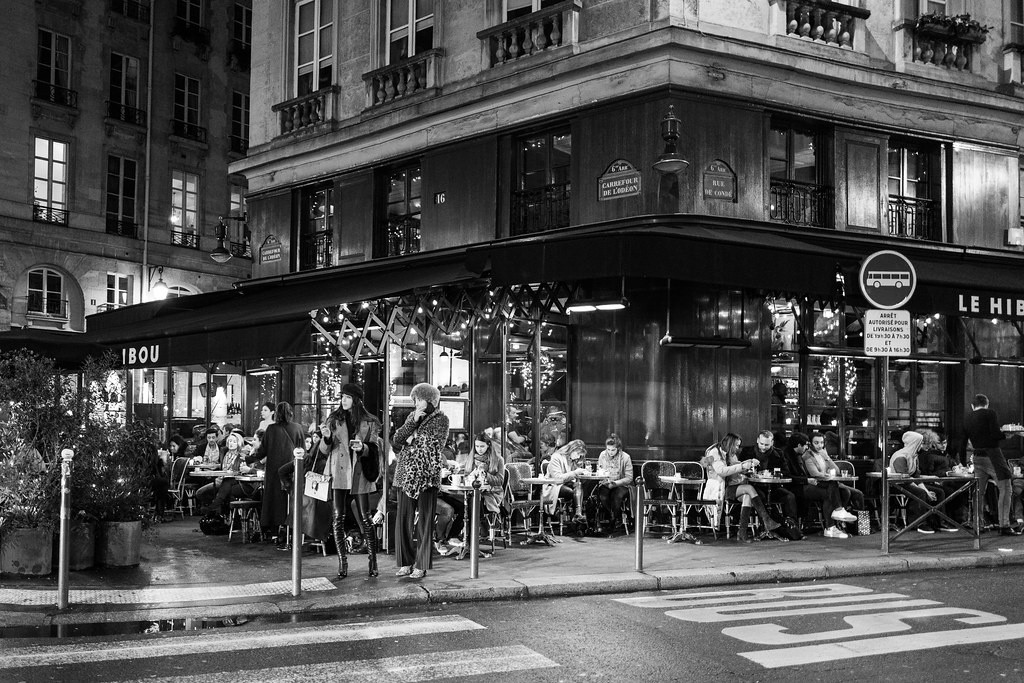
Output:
[703,429,958,544]
[526,433,633,538]
[456,432,505,538]
[169,401,323,551]
[392,383,455,579]
[960,394,1021,536]
[319,384,381,579]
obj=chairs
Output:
[150,451,1024,543]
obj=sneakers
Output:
[824,525,849,539]
[939,522,958,531]
[917,523,934,534]
[831,507,857,522]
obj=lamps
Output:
[209,213,247,263]
[149,265,168,300]
[651,104,690,172]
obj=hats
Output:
[410,383,440,409]
[340,383,364,400]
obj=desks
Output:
[189,470,231,518]
[517,479,566,547]
[444,485,492,560]
[576,474,614,538]
[661,477,706,545]
[814,474,859,537]
[748,475,793,542]
[865,471,910,532]
[949,471,975,528]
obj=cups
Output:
[256,470,264,478]
[194,467,200,473]
[448,474,461,487]
[674,473,681,479]
[1013,467,1020,476]
[774,467,780,475]
[584,460,591,476]
[841,470,848,478]
[189,461,193,466]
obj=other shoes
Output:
[608,520,617,534]
[575,524,584,536]
[479,517,490,537]
[1001,525,1022,536]
[395,566,413,577]
[975,528,991,536]
[448,538,464,548]
[271,537,279,545]
[572,515,586,525]
[155,516,172,523]
[434,540,451,555]
[613,524,621,533]
[410,569,427,579]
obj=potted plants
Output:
[0,344,160,576]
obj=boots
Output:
[737,504,753,543]
[331,513,348,580]
[358,514,379,577]
[751,496,781,531]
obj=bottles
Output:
[774,471,783,479]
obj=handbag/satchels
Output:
[303,468,334,502]
[774,511,801,540]
[199,511,230,536]
[499,499,510,515]
